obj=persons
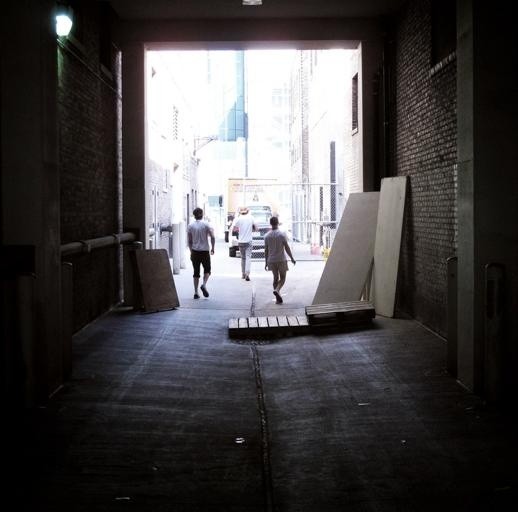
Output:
[230,206,258,282]
[262,216,296,303]
[185,207,215,299]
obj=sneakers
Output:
[242,274,250,281]
[272,289,283,304]
[194,294,200,299]
[200,285,209,297]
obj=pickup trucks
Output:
[228,205,272,257]
